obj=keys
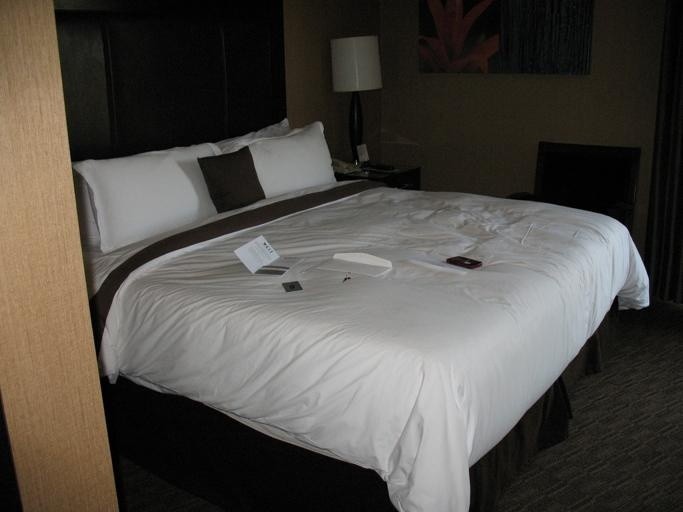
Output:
[342,277,351,283]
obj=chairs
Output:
[505,135,645,226]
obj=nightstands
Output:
[336,160,422,193]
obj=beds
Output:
[66,117,658,512]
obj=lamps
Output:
[326,33,385,164]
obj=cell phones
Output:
[446,255,482,269]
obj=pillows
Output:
[233,118,337,197]
[217,116,293,154]
[197,145,268,216]
[72,137,225,254]
[74,172,100,252]
